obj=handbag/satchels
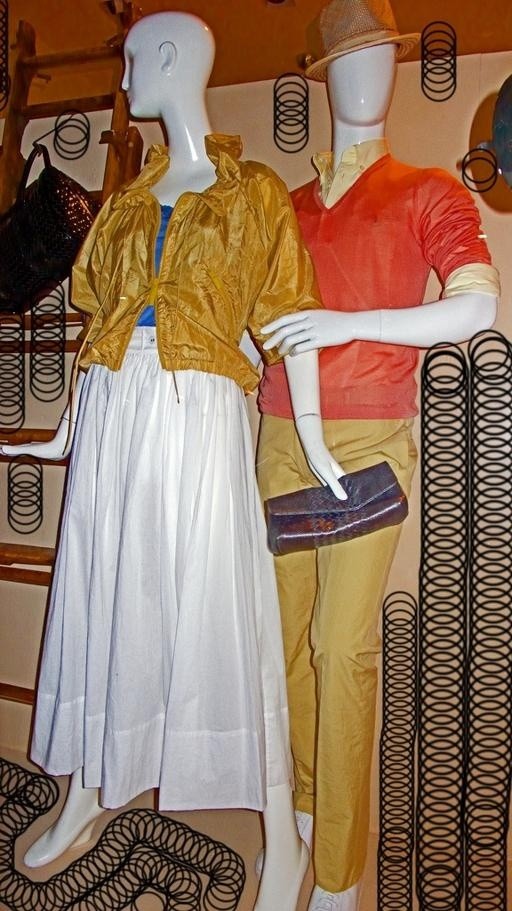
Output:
[0,143,94,316]
[264,460,408,559]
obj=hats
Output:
[306,2,422,82]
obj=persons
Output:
[2,9,359,911]
[234,0,503,911]
